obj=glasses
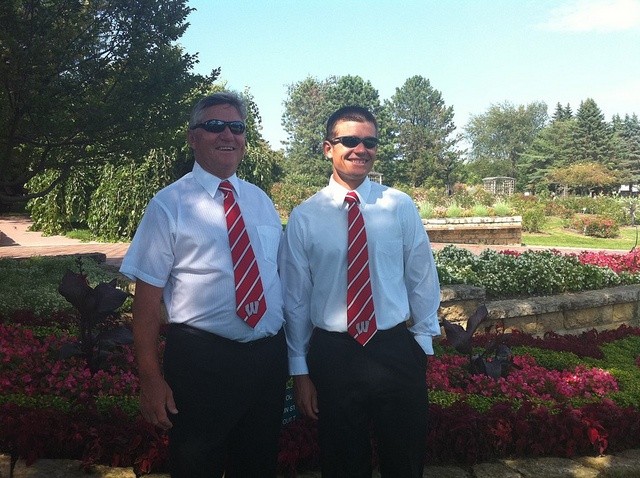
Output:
[331,136,378,149]
[190,120,246,135]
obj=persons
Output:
[278,105,442,477]
[119,94,287,477]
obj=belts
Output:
[180,326,284,348]
[317,323,406,342]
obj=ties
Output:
[345,192,378,347]
[217,182,267,328]
